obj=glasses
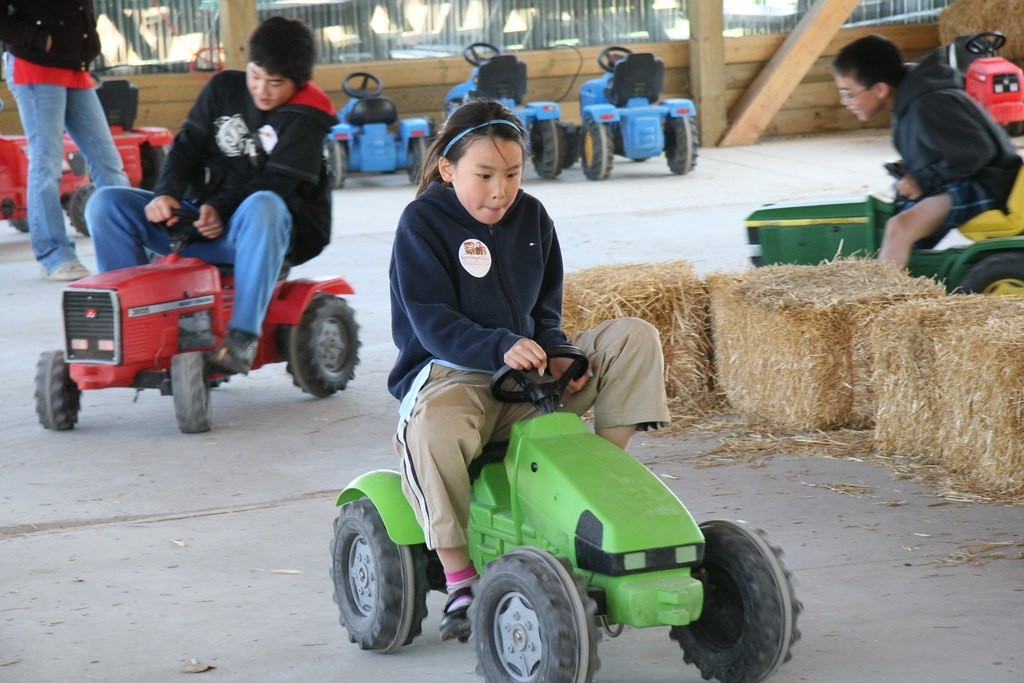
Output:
[838,88,867,99]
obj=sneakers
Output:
[40,258,89,282]
[206,329,259,375]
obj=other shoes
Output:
[439,586,472,641]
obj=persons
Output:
[0,0,132,280]
[83,15,340,376]
[386,102,671,645]
[830,34,1019,273]
